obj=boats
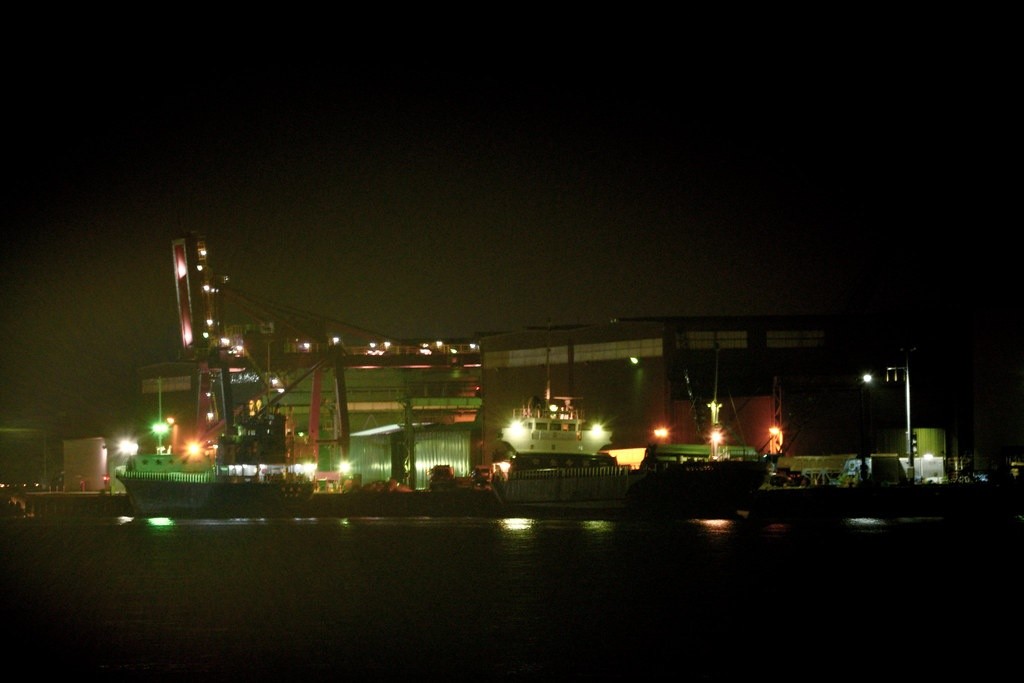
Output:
[836,480,980,518]
[494,459,765,518]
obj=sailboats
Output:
[116,274,314,519]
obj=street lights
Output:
[886,366,914,483]
